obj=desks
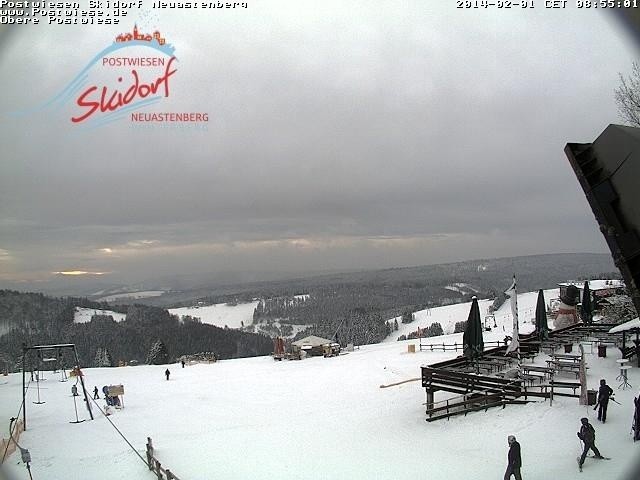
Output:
[440,327,636,405]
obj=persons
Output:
[181,359,185,368]
[577,379,613,468]
[93,386,100,399]
[504,435,522,480]
[165,368,170,380]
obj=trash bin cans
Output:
[588,390,598,405]
[564,343,572,353]
[598,345,607,357]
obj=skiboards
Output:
[577,455,611,472]
[632,395,640,443]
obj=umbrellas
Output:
[535,289,548,343]
[463,298,484,372]
[580,281,593,327]
